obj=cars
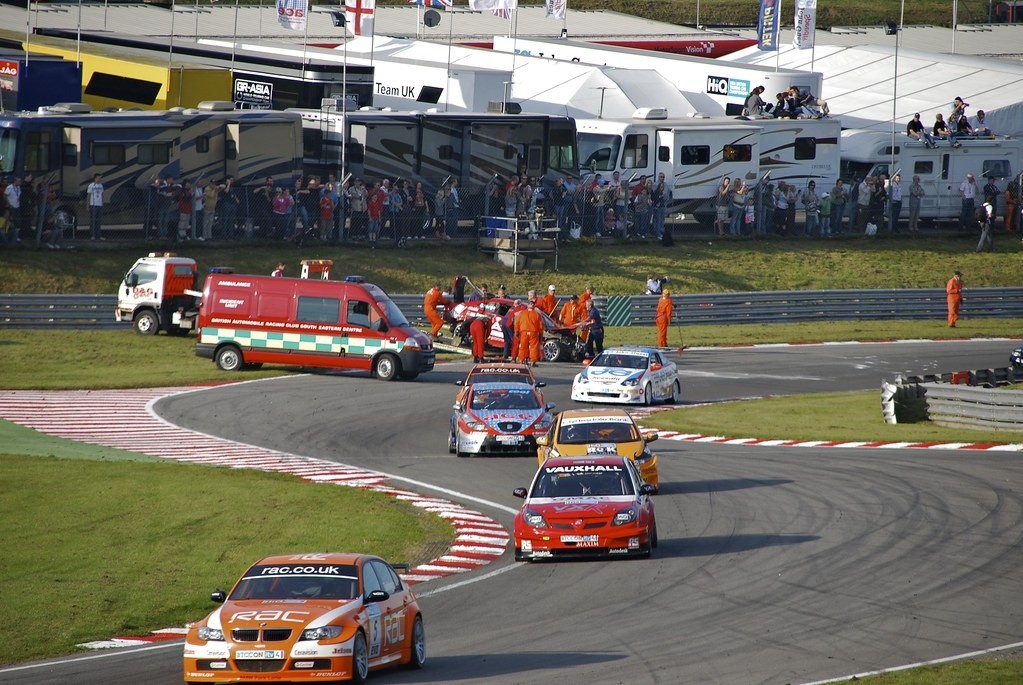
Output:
[448,384,556,458]
[513,456,657,562]
[184,553,425,685]
[536,409,659,495]
[570,347,681,407]
[441,276,595,361]
[455,357,547,403]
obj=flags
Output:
[275,0,306,30]
[794,0,816,49]
[545,0,565,20]
[410,0,452,7]
[469,0,518,12]
[345,0,374,37]
[494,9,511,18]
[757,0,779,51]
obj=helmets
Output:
[597,179,605,184]
[599,473,619,490]
[290,578,321,599]
[509,394,523,404]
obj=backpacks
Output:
[975,204,991,222]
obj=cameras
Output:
[962,103,969,106]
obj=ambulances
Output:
[196,276,435,381]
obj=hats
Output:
[587,285,596,291]
[988,176,997,180]
[956,271,963,276]
[549,284,555,290]
[498,284,505,290]
[955,97,962,103]
[570,295,577,300]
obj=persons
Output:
[717,174,925,239]
[907,97,991,148]
[271,262,285,277]
[742,85,827,120]
[946,271,963,327]
[87,173,106,241]
[646,273,671,294]
[253,177,460,247]
[485,163,669,240]
[423,276,605,367]
[0,173,76,248]
[656,289,672,350]
[143,174,240,241]
[959,163,1023,253]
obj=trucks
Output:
[114,253,472,358]
[0,99,597,255]
[842,130,1023,223]
[564,106,842,224]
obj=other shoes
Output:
[432,339,443,343]
[472,352,538,368]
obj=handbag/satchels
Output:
[865,222,877,235]
[569,222,581,239]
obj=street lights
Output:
[503,82,514,114]
[597,87,608,118]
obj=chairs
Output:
[55,212,75,238]
